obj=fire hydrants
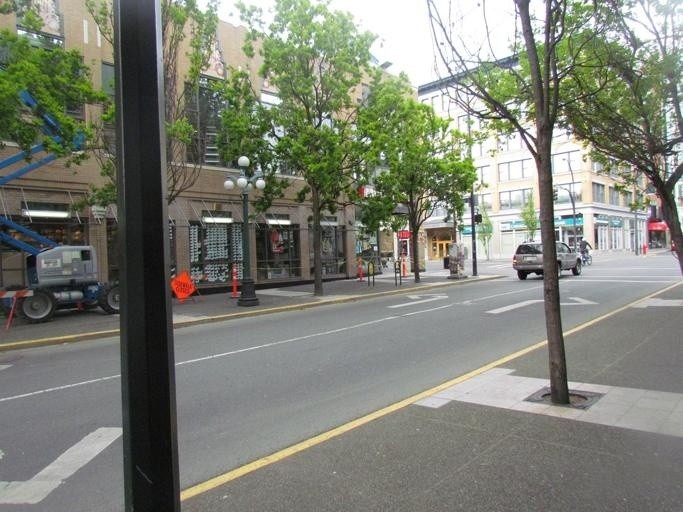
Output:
[641,244,647,255]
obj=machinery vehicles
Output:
[1,83,121,325]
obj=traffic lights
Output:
[552,188,558,201]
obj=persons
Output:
[579,236,592,262]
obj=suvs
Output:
[512,240,582,280]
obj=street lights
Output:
[561,158,577,252]
[224,154,269,306]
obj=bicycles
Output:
[580,249,591,265]
